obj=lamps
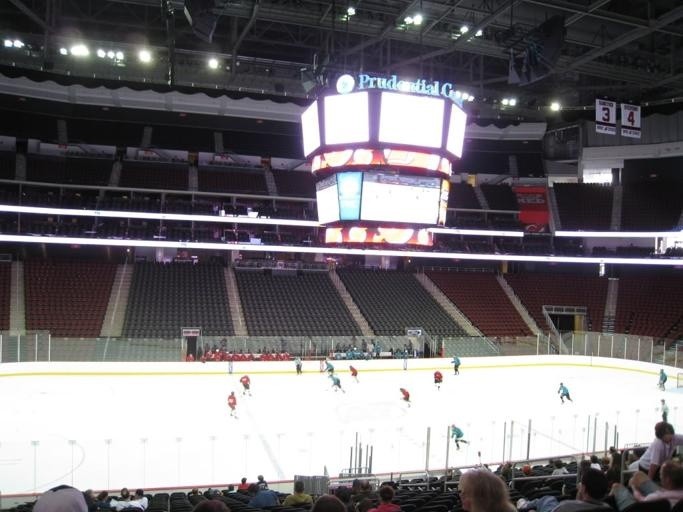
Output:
[297,0,471,228]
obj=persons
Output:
[399,388,411,404]
[658,368,667,391]
[34,476,314,511]
[497,422,681,511]
[558,382,573,403]
[322,359,333,376]
[432,371,444,391]
[451,424,470,450]
[348,363,358,382]
[660,398,669,422]
[450,355,463,376]
[314,479,400,510]
[326,374,344,392]
[239,374,250,395]
[184,336,419,373]
[228,391,237,418]
[459,469,516,512]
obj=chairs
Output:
[1,136,683,253]
[1,256,682,360]
[17,442,682,511]
